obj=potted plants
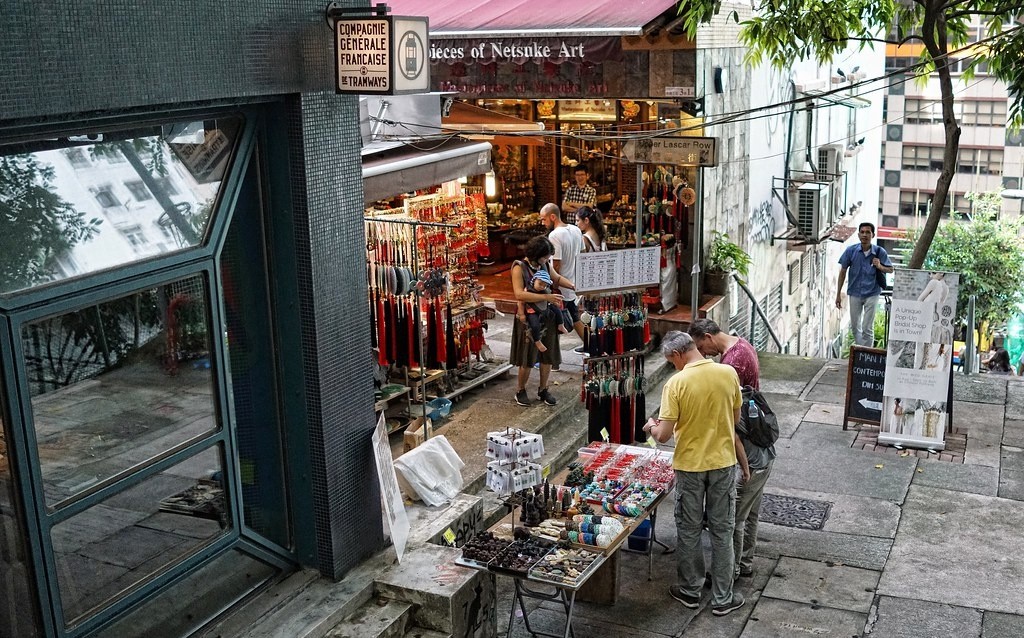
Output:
[703,231,754,295]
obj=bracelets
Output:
[570,284,574,290]
[648,424,657,433]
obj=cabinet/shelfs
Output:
[365,97,686,441]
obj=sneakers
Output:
[536,386,556,406]
[712,593,745,616]
[514,389,531,406]
[668,586,699,608]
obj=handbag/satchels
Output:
[876,246,886,290]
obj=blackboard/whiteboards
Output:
[844,345,887,426]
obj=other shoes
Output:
[573,347,590,358]
[706,572,712,581]
[740,570,753,577]
[534,363,559,371]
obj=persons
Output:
[836,223,894,348]
[534,203,589,371]
[957,347,967,372]
[703,431,775,584]
[575,206,608,253]
[562,164,597,234]
[686,319,760,582]
[517,270,568,352]
[936,344,946,372]
[890,398,904,434]
[642,331,744,616]
[509,235,576,407]
[981,347,1024,377]
[916,272,948,317]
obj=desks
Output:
[454,441,679,638]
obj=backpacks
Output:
[741,385,779,448]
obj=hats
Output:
[529,270,552,287]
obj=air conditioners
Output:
[818,177,844,223]
[818,143,844,188]
[770,175,832,247]
